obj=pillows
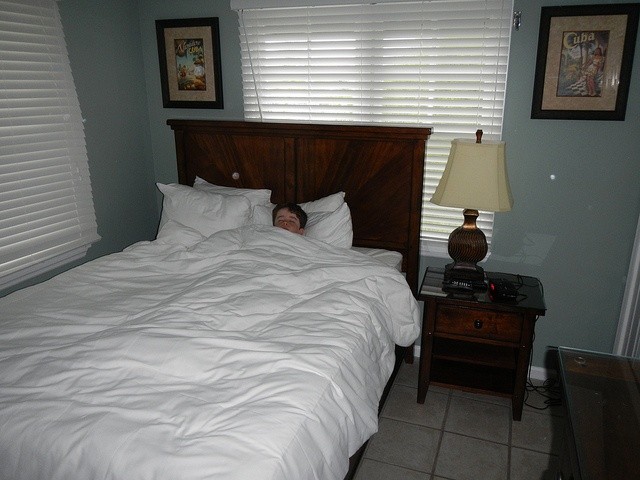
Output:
[253,202,354,250]
[154,182,250,240]
[192,176,272,225]
[266,190,346,212]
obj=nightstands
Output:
[416,266,548,421]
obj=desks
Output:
[558,346,640,480]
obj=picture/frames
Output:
[530,3,640,122]
[154,16,225,110]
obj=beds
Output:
[0,118,433,479]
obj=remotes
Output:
[442,281,473,290]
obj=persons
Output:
[174,37,206,91]
[583,48,605,96]
[273,201,307,235]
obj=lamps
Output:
[429,129,516,290]
